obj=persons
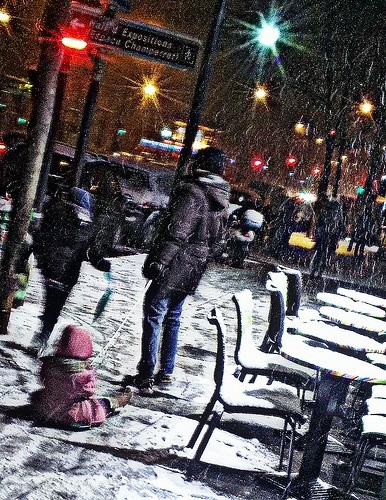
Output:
[0,132,29,197]
[310,196,374,280]
[33,324,132,427]
[126,146,231,390]
[34,186,111,344]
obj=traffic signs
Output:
[63,4,200,73]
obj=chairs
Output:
[189,267,320,483]
[343,352,386,500]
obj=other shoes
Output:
[154,370,173,385]
[122,375,154,391]
[112,387,132,407]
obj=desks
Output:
[260,344,386,500]
[279,286,386,452]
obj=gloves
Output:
[142,260,166,280]
[97,259,111,272]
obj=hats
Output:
[57,325,92,358]
[66,186,91,210]
[191,147,225,175]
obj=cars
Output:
[0,136,177,257]
[225,179,386,267]
